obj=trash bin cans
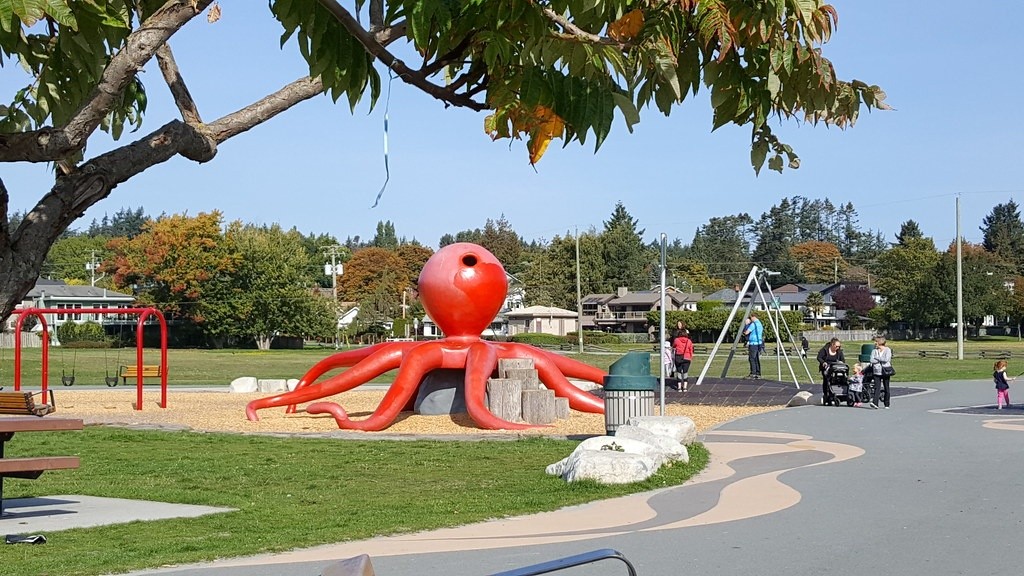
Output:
[858,344,879,370]
[603,353,657,436]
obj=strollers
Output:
[824,363,856,407]
[858,367,887,403]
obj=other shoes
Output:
[870,403,878,409]
[744,373,757,380]
[683,388,687,392]
[756,373,762,380]
[998,405,1003,410]
[1006,404,1013,408]
[853,402,863,406]
[678,389,683,393]
[884,404,890,409]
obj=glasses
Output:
[834,345,841,348]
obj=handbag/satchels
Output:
[675,355,684,364]
[801,346,806,355]
[882,367,896,376]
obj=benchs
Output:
[0,387,56,417]
[120,365,168,385]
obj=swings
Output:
[101,313,124,387]
[58,313,82,387]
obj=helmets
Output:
[665,341,670,347]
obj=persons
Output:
[817,338,845,403]
[870,338,891,409]
[672,330,693,392]
[670,319,690,377]
[849,362,865,407]
[665,341,671,376]
[802,337,808,358]
[743,313,763,379]
[992,361,1015,408]
